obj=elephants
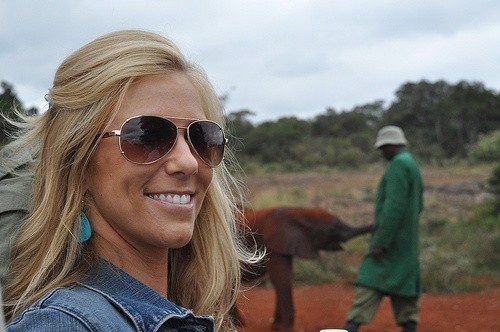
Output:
[228,206,378,332]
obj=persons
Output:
[341,126,424,331]
[0,30,269,332]
